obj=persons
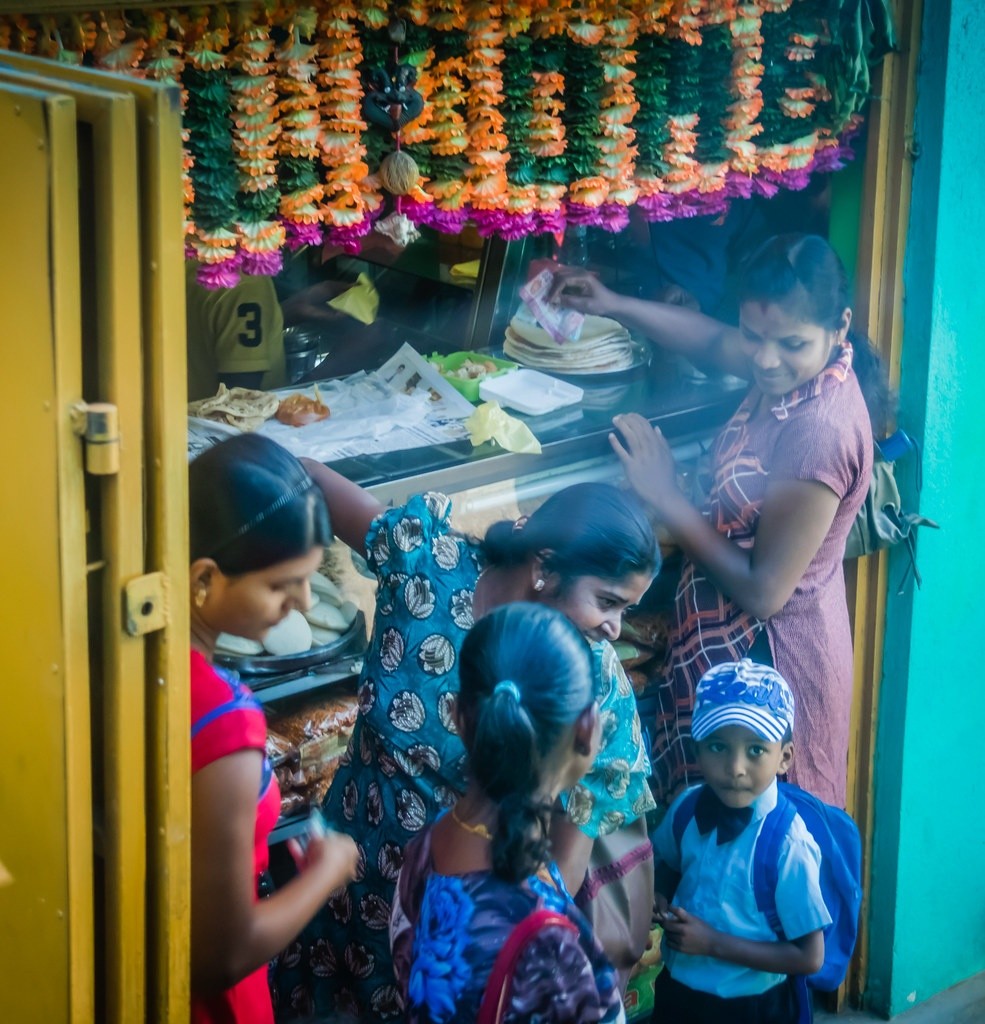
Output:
[389,601,626,1024]
[273,457,663,1024]
[649,660,825,1024]
[190,433,361,1023]
[543,232,876,812]
[185,258,362,403]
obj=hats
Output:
[690,658,796,743]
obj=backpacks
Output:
[672,780,861,991]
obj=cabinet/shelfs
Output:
[186,338,755,852]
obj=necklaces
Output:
[451,803,555,887]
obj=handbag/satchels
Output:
[844,429,938,592]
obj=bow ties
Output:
[694,785,753,846]
